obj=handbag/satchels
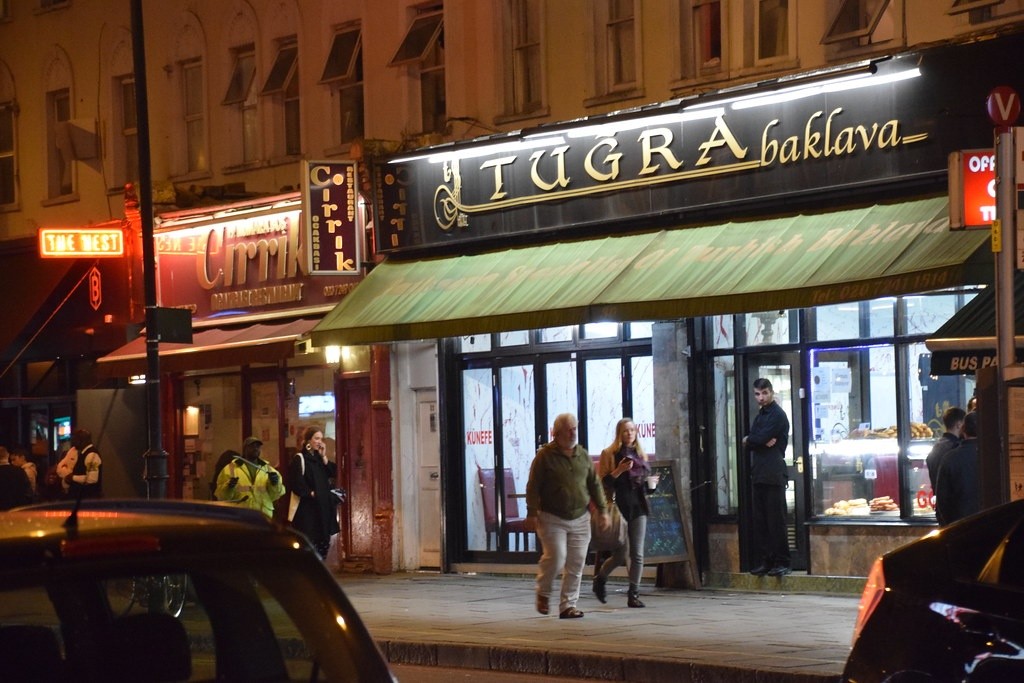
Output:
[589,500,628,552]
[287,453,304,522]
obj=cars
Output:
[842,499,1024,682]
[0,498,398,683]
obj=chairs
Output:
[478,466,532,553]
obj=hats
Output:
[243,437,263,449]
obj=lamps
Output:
[869,55,893,73]
[182,405,200,465]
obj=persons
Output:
[592,418,660,608]
[214,436,287,520]
[742,377,794,577]
[924,395,991,530]
[285,425,346,559]
[524,413,611,618]
[0,427,103,512]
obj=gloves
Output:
[228,476,239,490]
[269,471,279,485]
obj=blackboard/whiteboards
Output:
[644,459,694,565]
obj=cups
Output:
[647,476,659,489]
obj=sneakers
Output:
[591,575,607,604]
[750,565,771,577]
[628,593,646,608]
[767,566,792,577]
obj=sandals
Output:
[536,594,549,615]
[560,607,584,618]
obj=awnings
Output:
[309,184,992,348]
[0,249,91,404]
[96,308,322,380]
